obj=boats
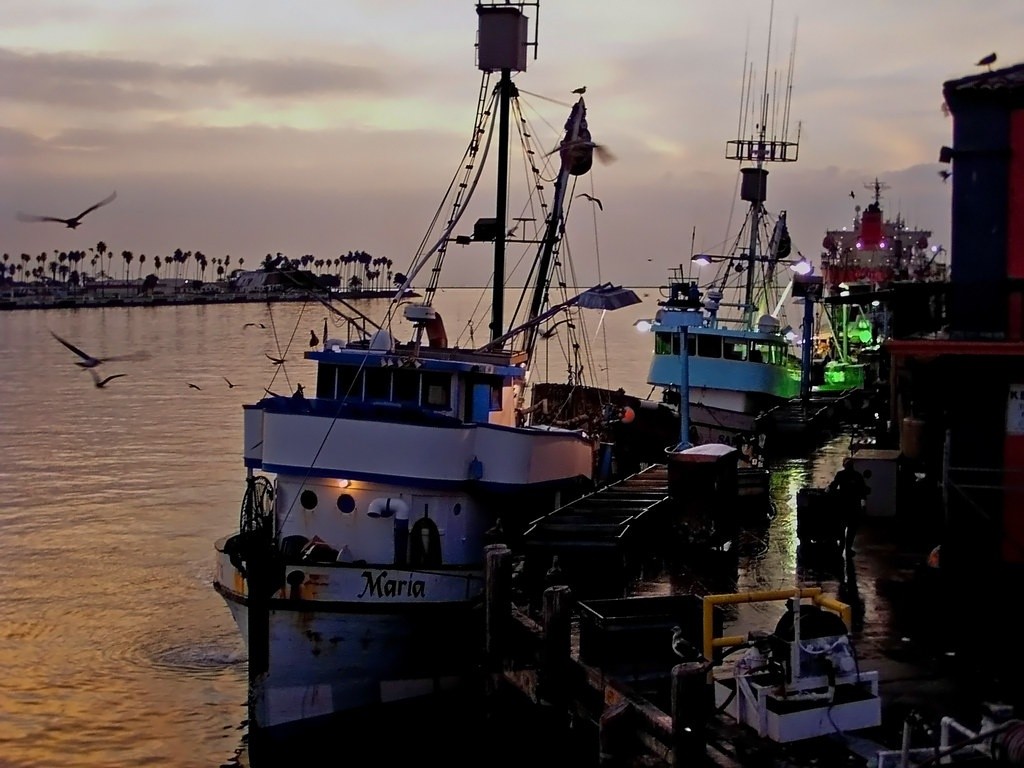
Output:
[804,174,949,345]
[645,1,881,464]
[213,0,699,767]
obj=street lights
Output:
[631,318,696,454]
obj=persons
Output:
[823,458,865,558]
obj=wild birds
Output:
[975,52,997,72]
[49,331,153,371]
[89,369,128,389]
[574,194,603,211]
[849,190,855,199]
[264,382,306,398]
[223,376,242,388]
[243,323,267,330]
[938,170,951,184]
[309,330,319,352]
[16,190,118,230]
[186,382,201,390]
[265,353,288,365]
